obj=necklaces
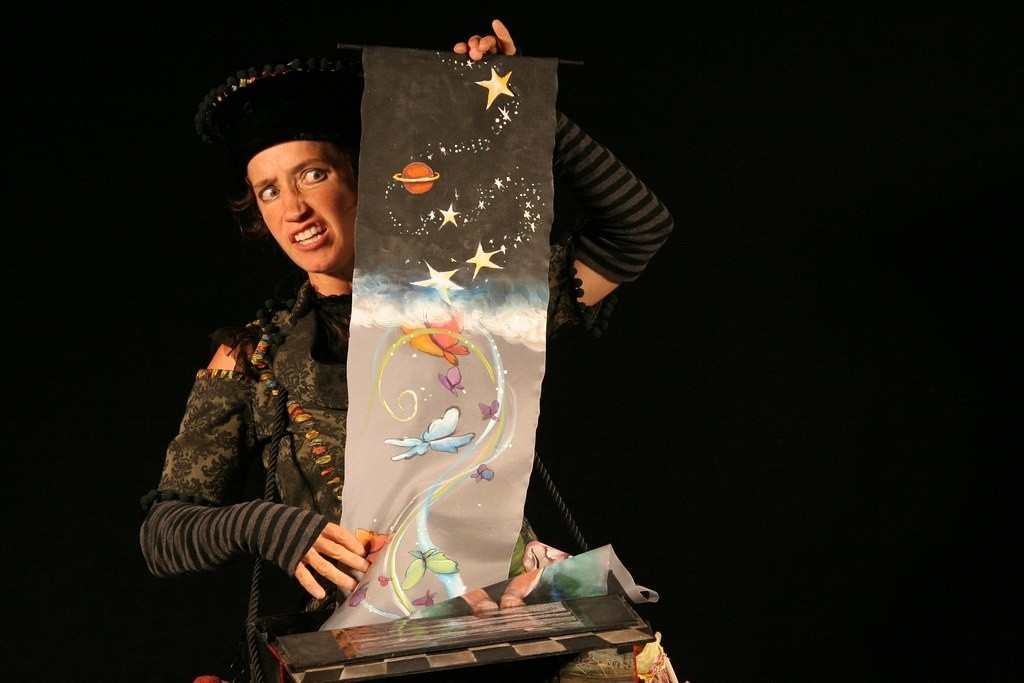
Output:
[253,291,346,496]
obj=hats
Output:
[194,48,361,179]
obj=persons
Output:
[139,19,677,683]
